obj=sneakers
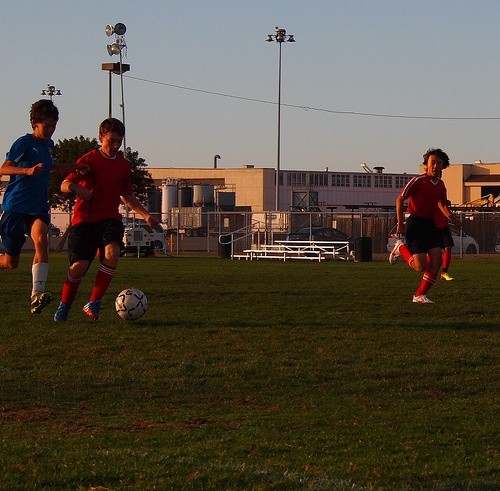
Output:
[413,294,434,303]
[389,240,404,265]
[31,292,52,314]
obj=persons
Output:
[54,117,157,322]
[389,149,462,303]
[0,99,90,314]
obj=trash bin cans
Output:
[218,235,231,258]
[354,237,372,262]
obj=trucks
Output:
[120,217,166,250]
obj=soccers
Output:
[115,288,148,321]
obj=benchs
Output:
[230,241,351,262]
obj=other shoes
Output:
[82,300,101,321]
[54,301,71,321]
[441,273,454,281]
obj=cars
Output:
[285,228,353,257]
[386,223,479,253]
[48,224,60,237]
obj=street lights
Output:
[265,27,297,212]
[41,85,62,100]
